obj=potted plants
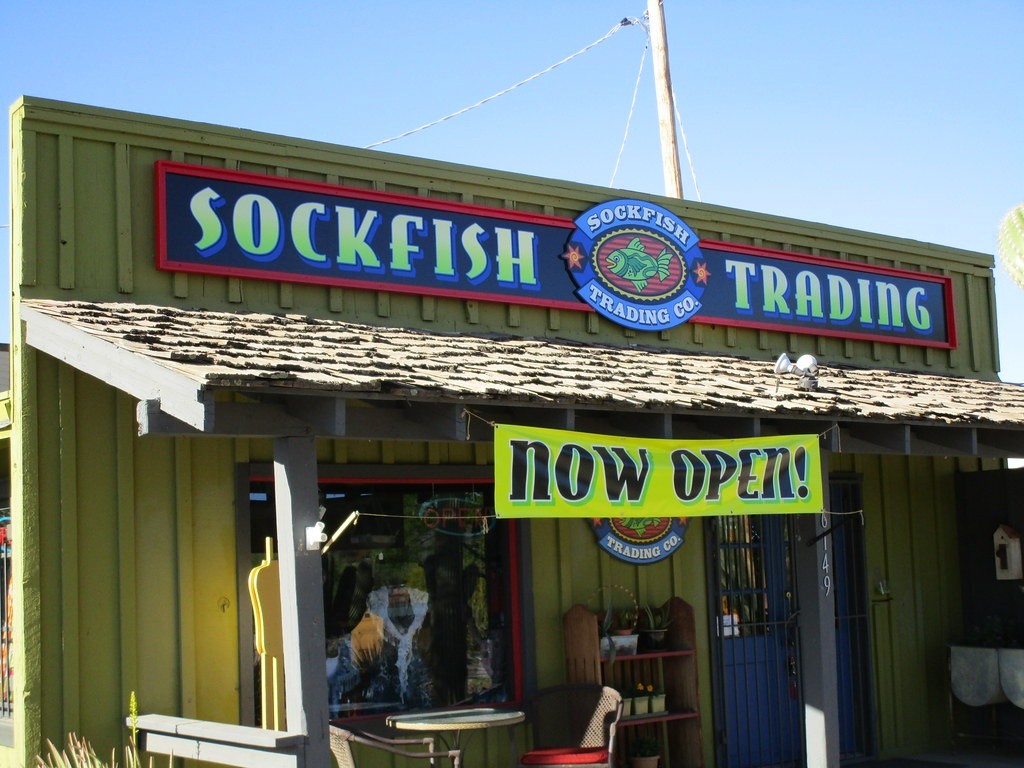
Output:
[604,606,638,635]
[613,685,633,717]
[628,734,661,768]
[636,598,675,649]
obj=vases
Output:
[651,695,666,713]
[633,697,649,715]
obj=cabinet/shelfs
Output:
[562,596,705,768]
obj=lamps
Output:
[774,353,818,376]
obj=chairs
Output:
[328,720,461,768]
[520,686,624,768]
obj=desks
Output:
[385,708,525,768]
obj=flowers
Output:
[633,683,664,697]
